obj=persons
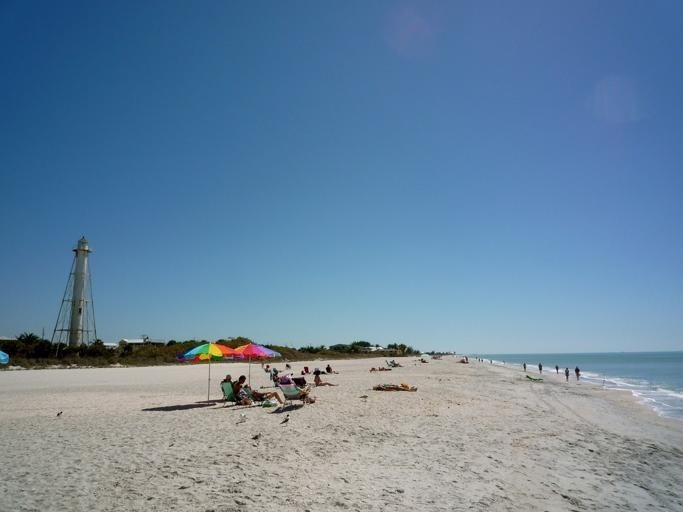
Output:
[575,366,581,381]
[295,384,315,403]
[565,367,570,381]
[313,370,339,386]
[326,364,339,374]
[538,362,542,374]
[219,365,296,404]
[523,362,526,371]
[555,365,559,374]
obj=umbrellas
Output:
[235,343,281,388]
[184,342,244,406]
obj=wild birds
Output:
[254,432,263,443]
[56,411,63,417]
[360,394,369,402]
[280,414,290,426]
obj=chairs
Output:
[220,377,314,411]
